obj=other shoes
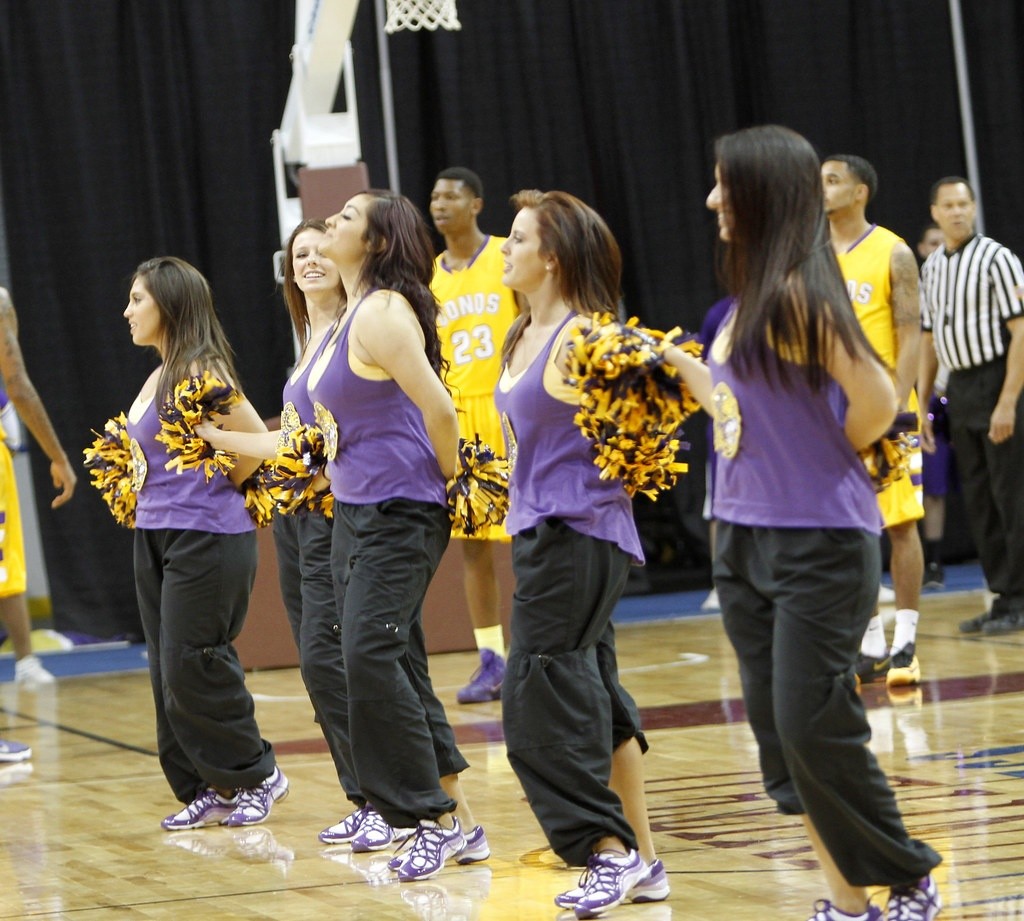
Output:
[699,587,720,611]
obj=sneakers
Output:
[388,815,491,883]
[456,648,506,703]
[868,873,943,921]
[853,652,890,684]
[806,899,885,921]
[317,805,418,853]
[227,765,290,827]
[14,658,56,686]
[554,849,671,920]
[878,588,896,603]
[959,611,1024,636]
[885,641,922,687]
[0,740,32,762]
[160,788,239,831]
[921,563,946,590]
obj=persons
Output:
[659,126,941,921]
[703,177,1024,687]
[0,286,78,763]
[193,168,670,919]
[113,257,289,831]
